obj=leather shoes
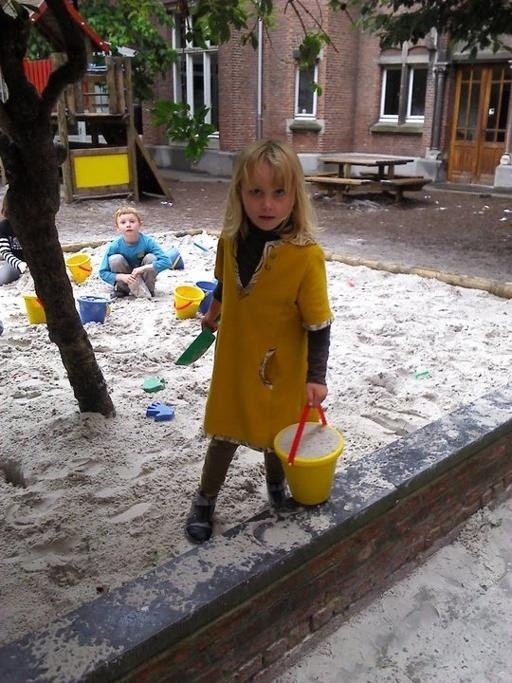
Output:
[265,451,285,487]
[185,487,215,542]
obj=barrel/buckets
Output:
[65,254,93,284]
[174,285,205,320]
[196,281,217,312]
[22,294,47,324]
[77,295,111,324]
[272,401,345,505]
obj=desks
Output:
[317,152,414,201]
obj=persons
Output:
[1,199,29,284]
[183,134,331,544]
[98,206,168,298]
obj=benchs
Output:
[380,176,432,206]
[304,171,339,177]
[305,176,371,201]
[359,170,425,187]
